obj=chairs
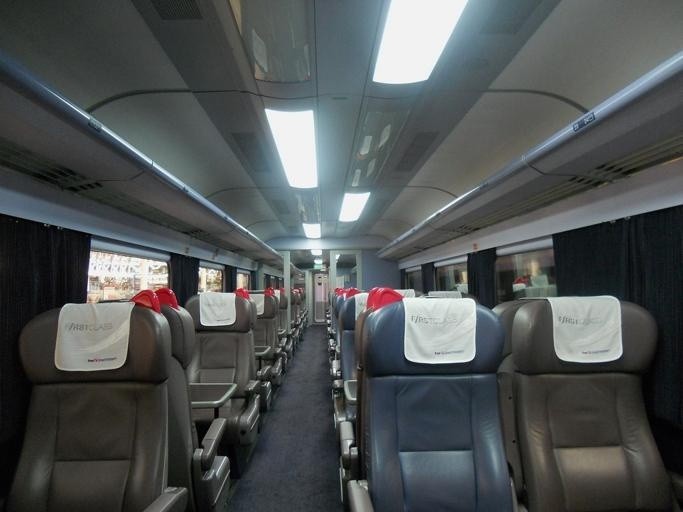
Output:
[329,284,682,512]
[0,286,308,512]
